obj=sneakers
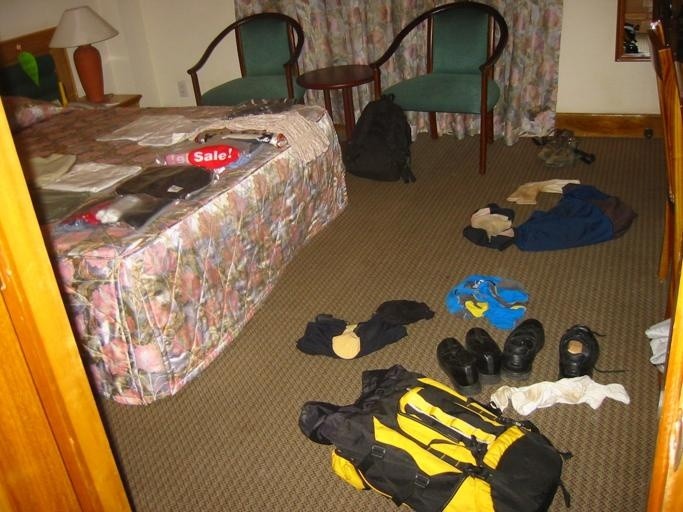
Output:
[502,318,545,380]
[558,324,600,381]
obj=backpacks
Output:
[344,94,411,182]
[297,364,574,512]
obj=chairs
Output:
[371,1,510,176]
[187,10,305,108]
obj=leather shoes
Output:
[466,327,502,385]
[437,337,480,394]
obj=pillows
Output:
[0,91,71,133]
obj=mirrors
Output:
[615,1,681,65]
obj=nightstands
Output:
[78,91,145,109]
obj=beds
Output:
[0,28,344,404]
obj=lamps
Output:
[46,3,120,104]
